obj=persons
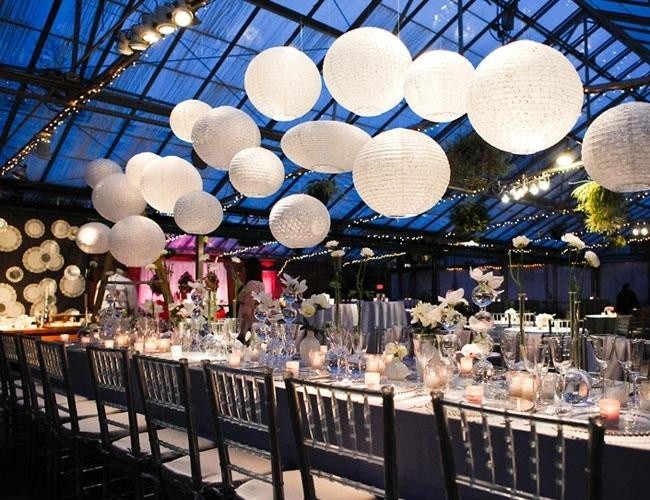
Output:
[616,283,640,315]
[236,256,265,347]
[215,305,226,319]
[93,263,138,311]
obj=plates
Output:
[0,217,87,330]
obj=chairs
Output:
[285,376,403,498]
[1,329,152,497]
[200,360,383,500]
[427,391,609,498]
[365,321,650,370]
[86,344,216,498]
[130,353,284,500]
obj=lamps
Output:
[280,118,372,178]
[102,1,204,67]
[320,22,412,121]
[401,46,475,124]
[107,212,166,270]
[350,125,455,222]
[462,35,589,159]
[170,96,264,172]
[171,189,225,239]
[262,192,336,253]
[227,144,289,200]
[241,42,323,125]
[74,220,112,257]
[579,98,649,195]
[88,150,207,224]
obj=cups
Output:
[308,351,325,369]
[285,361,299,379]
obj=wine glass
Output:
[588,336,649,422]
[544,335,576,416]
[325,326,410,390]
[80,314,297,371]
[411,332,551,426]
